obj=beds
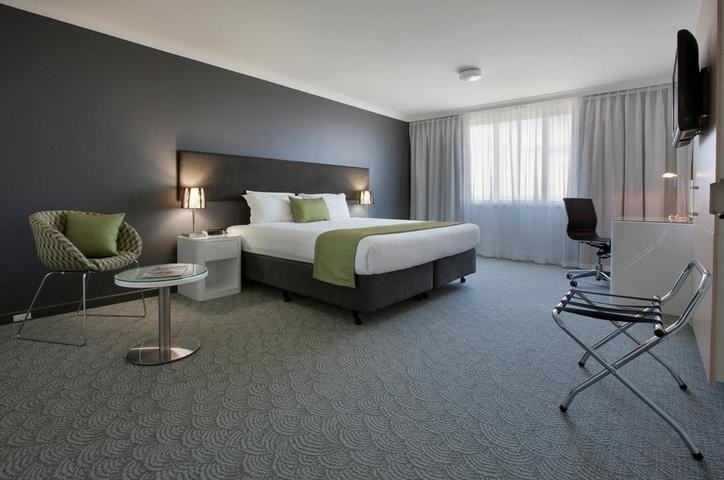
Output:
[222,217,483,326]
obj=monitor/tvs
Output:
[671,29,711,148]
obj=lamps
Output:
[459,70,482,82]
[662,172,688,218]
[180,187,207,237]
[358,190,374,218]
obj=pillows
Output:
[241,194,304,225]
[301,192,350,220]
[287,195,331,223]
[64,210,128,259]
[245,190,296,196]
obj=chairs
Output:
[562,198,611,288]
[15,210,147,347]
[551,256,714,460]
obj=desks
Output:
[113,262,209,365]
[609,215,691,319]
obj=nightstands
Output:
[176,235,242,302]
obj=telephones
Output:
[188,230,209,240]
